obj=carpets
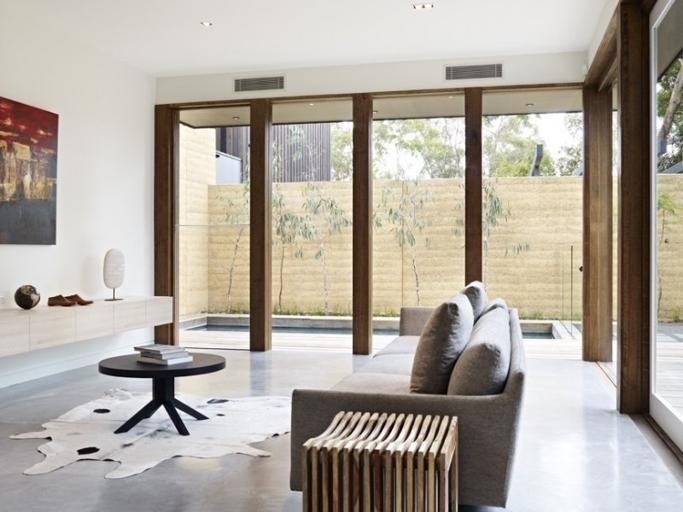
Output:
[8,389,292,478]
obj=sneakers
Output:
[65,293,93,306]
[47,294,77,307]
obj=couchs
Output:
[289,306,527,512]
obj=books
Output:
[134,343,193,366]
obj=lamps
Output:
[102,248,125,301]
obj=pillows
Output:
[447,306,510,396]
[462,280,487,320]
[409,293,473,393]
[476,297,510,322]
